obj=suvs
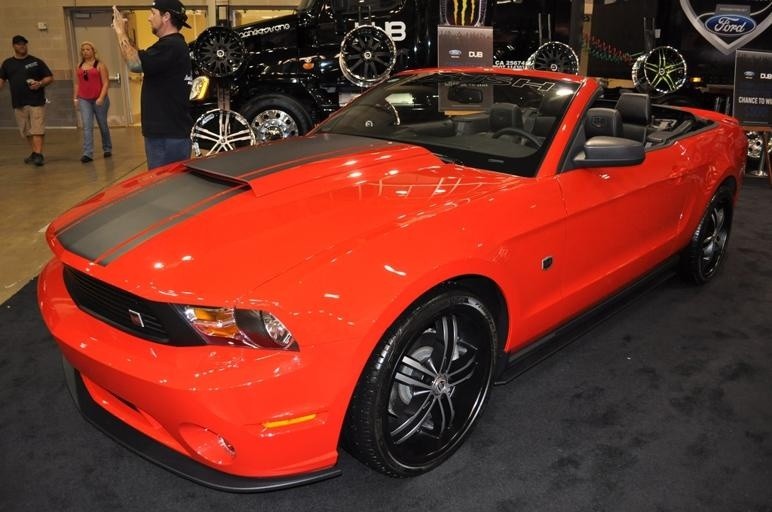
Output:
[188,0,573,145]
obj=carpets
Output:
[2,175,768,512]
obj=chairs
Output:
[525,94,572,149]
[583,108,625,140]
[615,92,650,145]
[482,102,523,143]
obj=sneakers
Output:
[104,152,112,157]
[81,156,91,162]
[34,153,43,165]
[24,152,34,163]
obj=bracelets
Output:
[73,98,79,99]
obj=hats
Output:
[13,35,27,43]
[153,0,192,28]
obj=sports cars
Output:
[37,65,749,493]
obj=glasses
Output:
[162,8,188,22]
[84,70,88,80]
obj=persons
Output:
[112,0,192,169]
[73,41,112,162]
[1,35,53,166]
